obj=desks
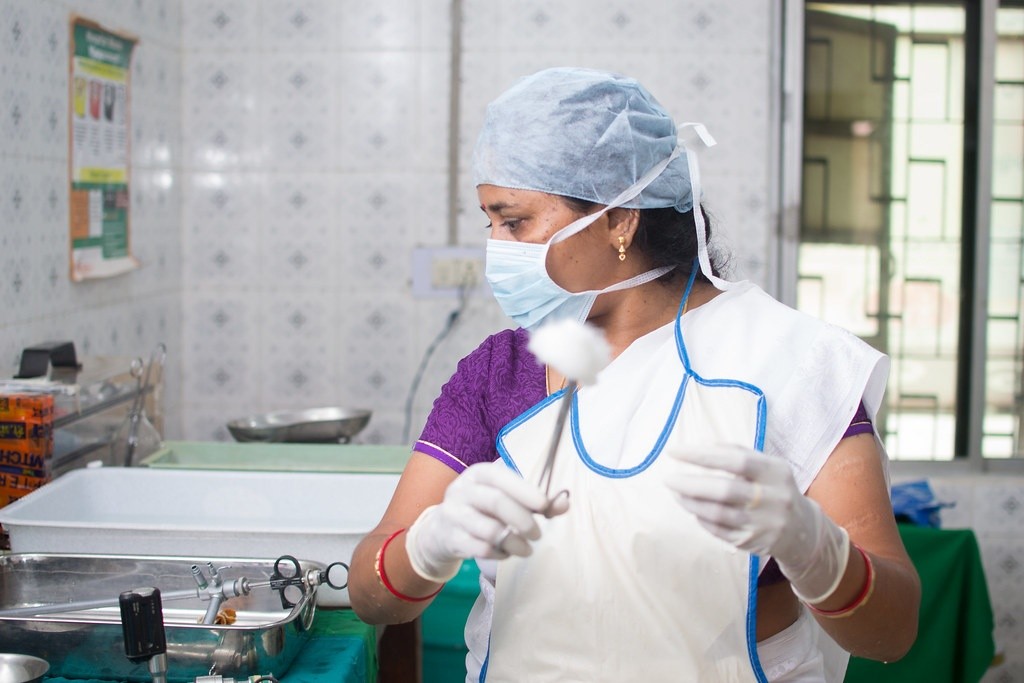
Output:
[42,608,422,683]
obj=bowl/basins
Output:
[0,653,50,683]
[227,405,372,443]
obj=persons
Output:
[348,66,922,683]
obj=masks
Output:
[483,120,640,332]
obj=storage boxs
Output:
[0,467,402,606]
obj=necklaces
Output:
[545,298,689,397]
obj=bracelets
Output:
[375,529,444,603]
[802,544,876,619]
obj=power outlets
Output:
[411,244,495,302]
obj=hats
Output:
[473,66,704,212]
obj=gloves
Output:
[667,441,849,604]
[405,462,570,582]
